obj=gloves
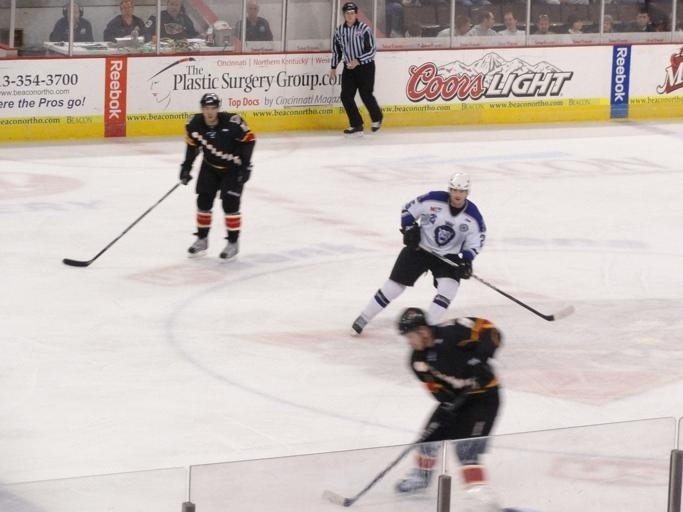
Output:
[456,256,473,280]
[180,166,192,185]
[404,228,420,249]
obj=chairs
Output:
[403,2,650,37]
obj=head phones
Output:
[63,3,83,16]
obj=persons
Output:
[350,174,487,336]
[235,0,274,42]
[146,0,205,45]
[373,0,683,40]
[205,21,236,46]
[396,308,503,499]
[328,3,383,133]
[179,92,256,260]
[104,0,145,44]
[48,2,95,53]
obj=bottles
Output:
[206,24,213,43]
[131,30,138,46]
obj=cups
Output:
[143,29,152,42]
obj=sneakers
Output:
[398,477,431,491]
[220,241,239,259]
[188,238,208,253]
[372,115,383,131]
[351,315,368,336]
[345,125,364,134]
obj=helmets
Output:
[200,93,220,107]
[342,2,358,14]
[398,308,425,335]
[448,171,470,192]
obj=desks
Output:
[43,36,235,56]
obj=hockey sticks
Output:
[63,180,182,267]
[321,419,438,507]
[419,242,573,321]
